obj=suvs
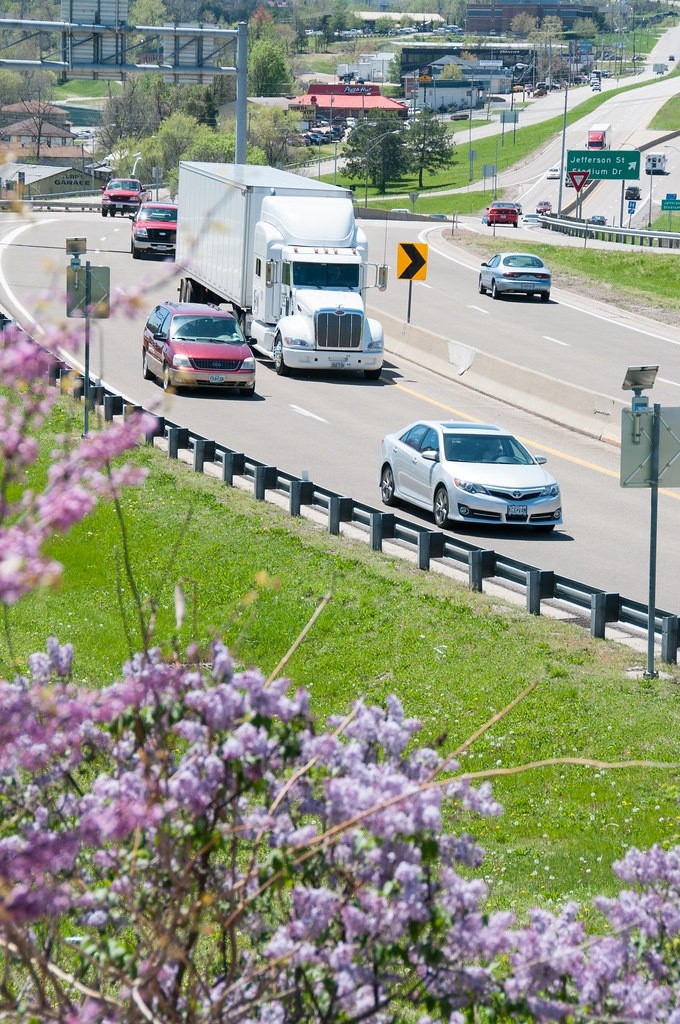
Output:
[625,186,642,200]
[129,202,177,261]
[142,302,256,396]
[100,177,147,216]
[536,82,560,90]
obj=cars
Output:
[589,216,607,224]
[590,77,601,91]
[485,202,518,228]
[546,168,561,179]
[535,201,553,213]
[286,125,346,147]
[378,419,563,530]
[477,253,552,300]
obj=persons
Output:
[483,440,501,459]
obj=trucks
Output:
[175,160,387,379]
[584,124,612,150]
[645,153,667,174]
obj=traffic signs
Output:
[567,149,640,180]
